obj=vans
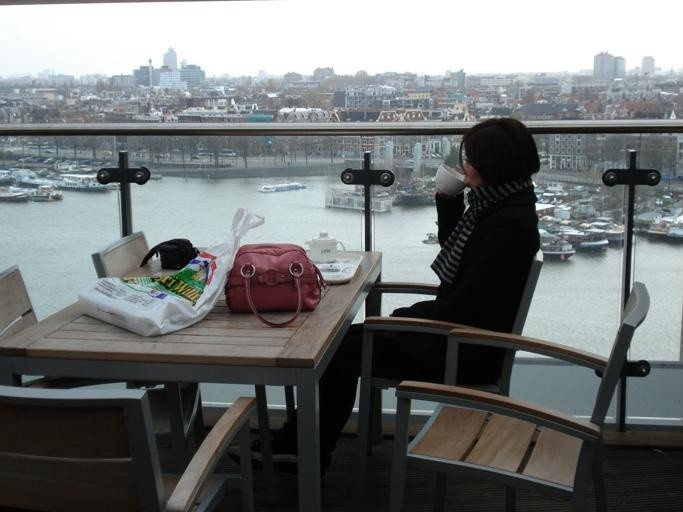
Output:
[104,150,111,155]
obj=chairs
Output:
[387,281,649,510]
[1,384,257,512]
[90,230,211,389]
[351,246,544,508]
[2,265,206,474]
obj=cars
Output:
[18,157,115,172]
[43,150,53,154]
[538,149,550,165]
[410,151,442,160]
[132,146,201,160]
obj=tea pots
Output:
[303,232,345,263]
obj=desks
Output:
[1,249,382,512]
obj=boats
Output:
[258,182,308,193]
[0,169,117,201]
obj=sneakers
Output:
[226,428,331,481]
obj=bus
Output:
[196,145,239,157]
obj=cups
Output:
[435,164,468,198]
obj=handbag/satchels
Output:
[155,239,199,271]
[226,244,327,328]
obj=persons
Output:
[227,118,540,474]
